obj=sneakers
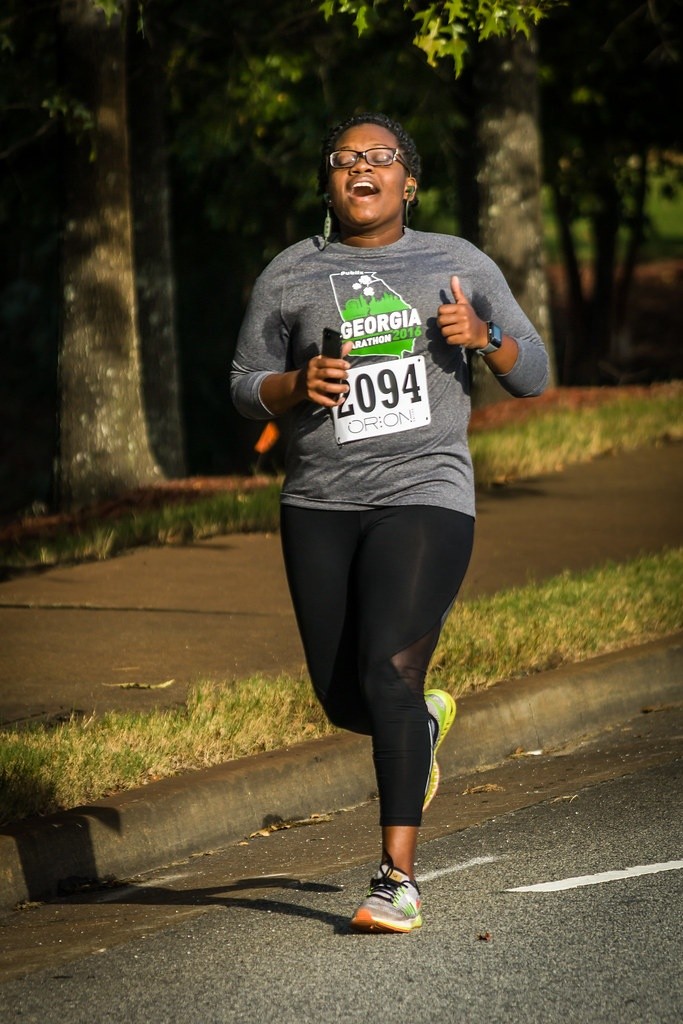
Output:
[425,688,459,812]
[352,864,424,932]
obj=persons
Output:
[229,113,552,933]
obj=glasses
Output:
[328,147,409,172]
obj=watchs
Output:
[473,319,503,355]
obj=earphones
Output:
[405,186,415,197]
[322,191,331,203]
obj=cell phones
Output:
[321,328,343,402]
